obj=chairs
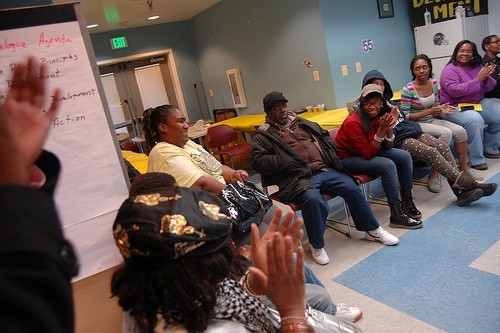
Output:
[207,124,253,171]
[120,141,136,151]
[261,91,489,237]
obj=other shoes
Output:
[427,175,442,193]
[471,162,488,170]
[461,170,484,181]
[484,151,500,158]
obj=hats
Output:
[264,91,289,113]
[112,185,233,262]
[361,83,383,97]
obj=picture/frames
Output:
[377,0,394,19]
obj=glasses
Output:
[490,40,500,44]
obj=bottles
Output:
[424,7,431,25]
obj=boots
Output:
[389,201,423,229]
[452,170,497,196]
[399,188,422,219]
[448,176,484,206]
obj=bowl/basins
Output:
[306,107,326,112]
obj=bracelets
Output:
[374,134,384,143]
[385,133,395,142]
[278,314,312,331]
[246,269,268,298]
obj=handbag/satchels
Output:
[216,178,272,236]
[267,303,362,333]
[392,120,422,148]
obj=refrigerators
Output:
[414,15,489,90]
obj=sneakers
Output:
[333,304,362,323]
[365,225,399,246]
[308,242,330,265]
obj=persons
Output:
[0,56,79,333]
[143,104,295,254]
[401,54,484,193]
[110,172,362,333]
[439,40,500,170]
[481,35,500,99]
[362,70,498,207]
[248,91,399,265]
[334,84,423,229]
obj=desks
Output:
[308,90,402,130]
[187,125,225,165]
[121,150,149,175]
[213,110,329,131]
